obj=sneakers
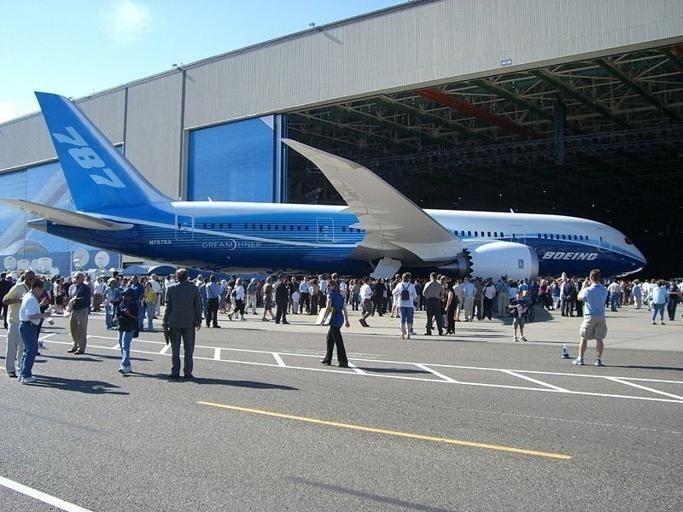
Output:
[119,366,131,374]
[572,358,584,364]
[228,312,290,324]
[514,336,517,342]
[402,325,455,340]
[68,349,77,352]
[8,369,36,383]
[184,373,193,377]
[521,337,527,340]
[168,373,179,378]
[595,359,601,366]
[75,350,84,354]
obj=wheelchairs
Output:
[508,300,535,323]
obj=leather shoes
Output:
[213,325,220,327]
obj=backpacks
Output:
[402,283,411,300]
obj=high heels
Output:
[321,359,331,365]
[338,363,348,367]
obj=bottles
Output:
[45,310,56,327]
[563,344,568,353]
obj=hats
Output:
[74,272,85,280]
[121,287,138,300]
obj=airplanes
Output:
[0,88,648,306]
